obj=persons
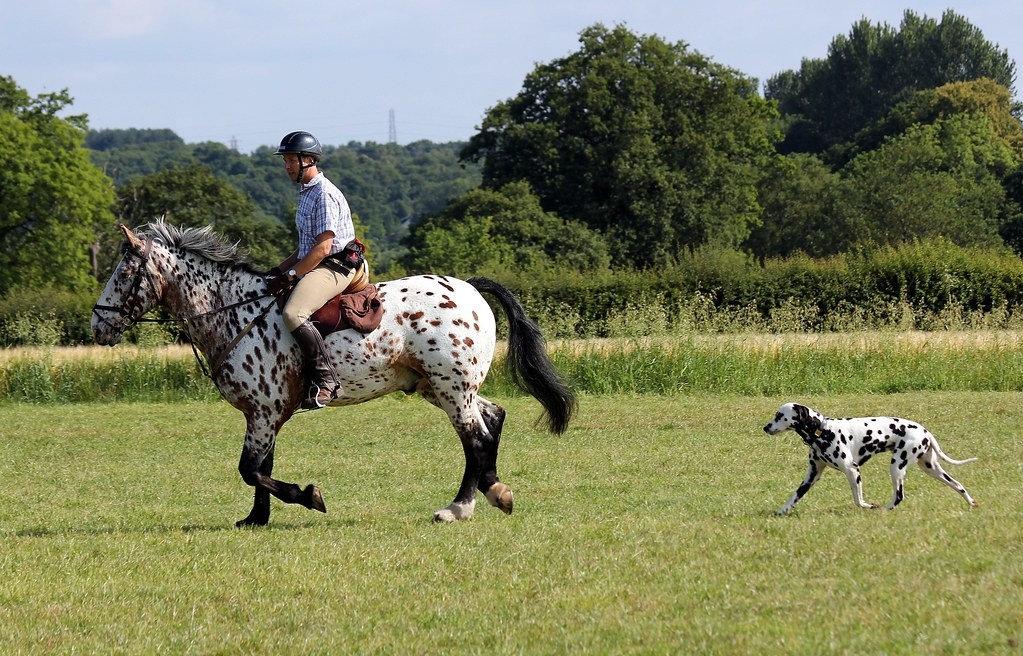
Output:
[267,131,370,409]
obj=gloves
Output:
[267,274,290,295]
[264,267,280,276]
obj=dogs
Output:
[765,403,983,511]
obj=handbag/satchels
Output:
[340,283,382,333]
[324,238,366,267]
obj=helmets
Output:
[273,131,321,162]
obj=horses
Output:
[92,216,579,528]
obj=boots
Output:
[290,320,344,410]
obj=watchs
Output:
[287,267,301,282]
